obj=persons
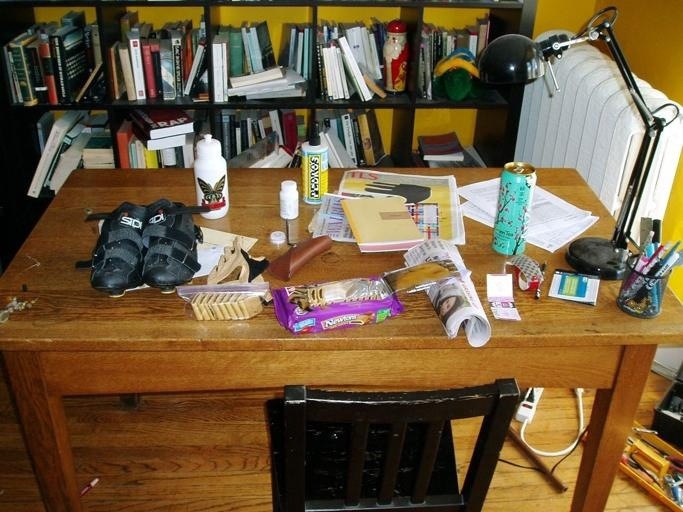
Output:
[435,294,464,327]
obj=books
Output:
[3,9,210,104]
[210,17,490,104]
[27,110,222,201]
[414,131,487,169]
[221,110,385,169]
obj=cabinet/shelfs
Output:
[1,0,539,209]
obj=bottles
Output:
[278,180,299,220]
[190,131,233,221]
[381,19,409,96]
[299,125,330,205]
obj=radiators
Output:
[512,26,683,253]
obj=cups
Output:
[616,254,672,319]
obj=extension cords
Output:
[516,387,544,422]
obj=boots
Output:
[75,201,149,298]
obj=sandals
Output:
[141,198,211,295]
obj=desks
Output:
[0,160,683,511]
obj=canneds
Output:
[490,160,537,256]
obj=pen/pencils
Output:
[79,476,102,497]
[618,231,681,317]
[536,260,547,298]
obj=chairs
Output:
[251,368,526,512]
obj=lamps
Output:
[467,4,677,285]
